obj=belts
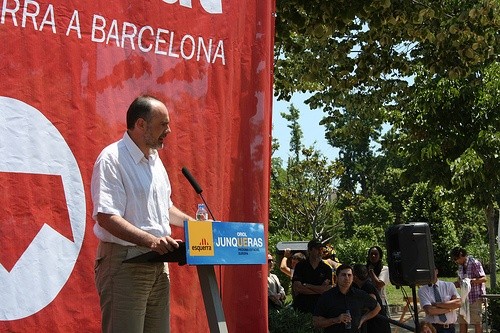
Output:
[433,323,453,329]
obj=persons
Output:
[417,265,464,333]
[321,244,343,285]
[311,264,392,333]
[292,238,337,317]
[351,263,388,318]
[265,252,287,311]
[450,246,487,333]
[90,95,220,333]
[280,248,307,286]
[353,246,390,328]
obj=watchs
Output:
[301,283,307,288]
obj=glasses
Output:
[268,259,273,264]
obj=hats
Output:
[308,239,327,250]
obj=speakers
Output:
[385,222,436,289]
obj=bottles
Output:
[345,310,351,329]
[196,203,208,221]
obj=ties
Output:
[434,284,447,323]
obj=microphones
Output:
[181,167,216,222]
